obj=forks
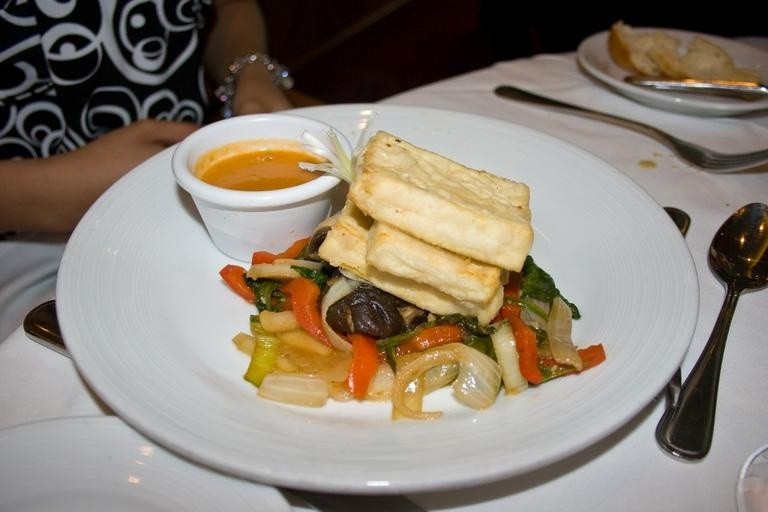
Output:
[494,84,764,175]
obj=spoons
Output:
[655,202,768,461]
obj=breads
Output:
[609,21,735,82]
[317,129,532,329]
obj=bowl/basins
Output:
[171,115,354,265]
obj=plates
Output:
[54,103,700,496]
[578,27,768,116]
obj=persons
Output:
[0,0,297,237]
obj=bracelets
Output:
[214,51,296,120]
